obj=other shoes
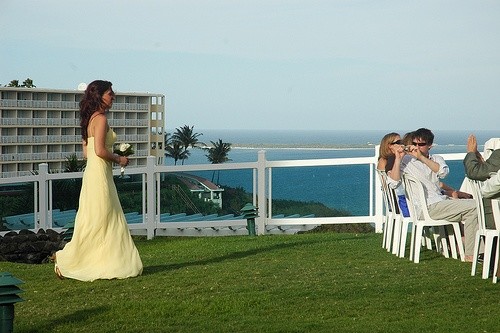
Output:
[390,140,403,145]
[465,253,473,262]
[478,253,484,261]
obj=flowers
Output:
[113,142,134,177]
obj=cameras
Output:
[402,146,410,152]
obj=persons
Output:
[49,79,134,279]
[377,127,500,277]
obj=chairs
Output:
[401,174,466,263]
[376,168,450,259]
[469,179,500,284]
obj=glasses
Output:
[412,142,427,146]
[104,91,115,96]
